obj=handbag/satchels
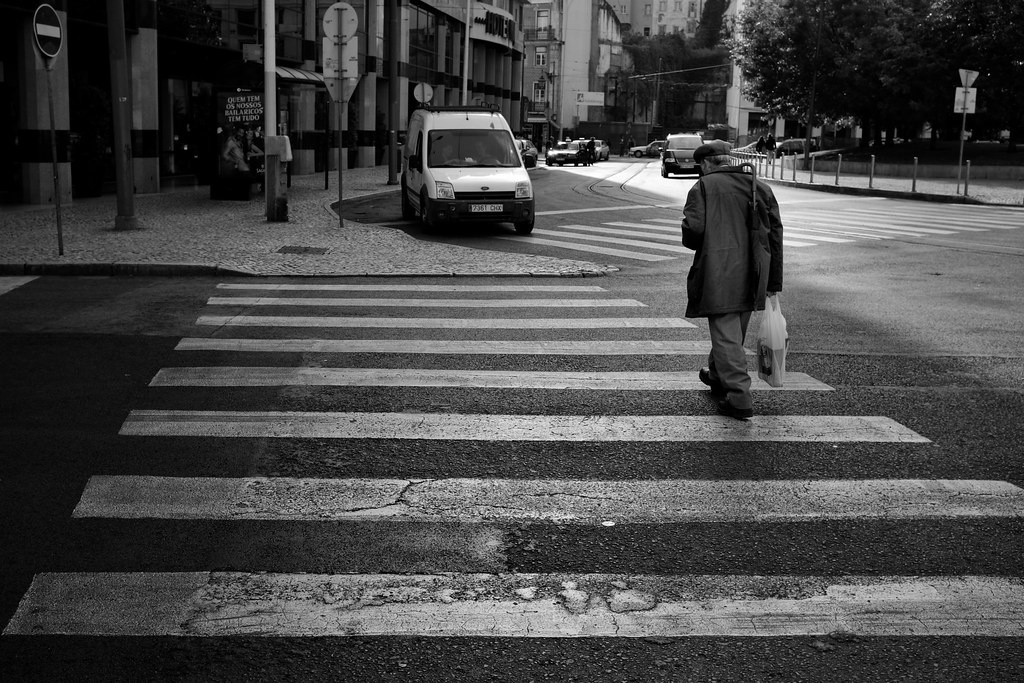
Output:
[758,293,789,386]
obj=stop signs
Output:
[34,3,63,57]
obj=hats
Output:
[693,139,732,164]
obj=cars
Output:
[515,139,538,169]
[658,133,705,178]
[629,140,668,157]
[546,139,610,167]
[730,138,817,159]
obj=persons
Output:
[221,121,266,183]
[545,136,555,164]
[681,138,784,419]
[765,133,776,165]
[605,134,636,158]
[756,136,767,163]
[586,136,596,166]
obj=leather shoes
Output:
[718,400,753,418]
[698,367,712,385]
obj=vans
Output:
[400,102,536,236]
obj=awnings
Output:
[239,58,326,84]
